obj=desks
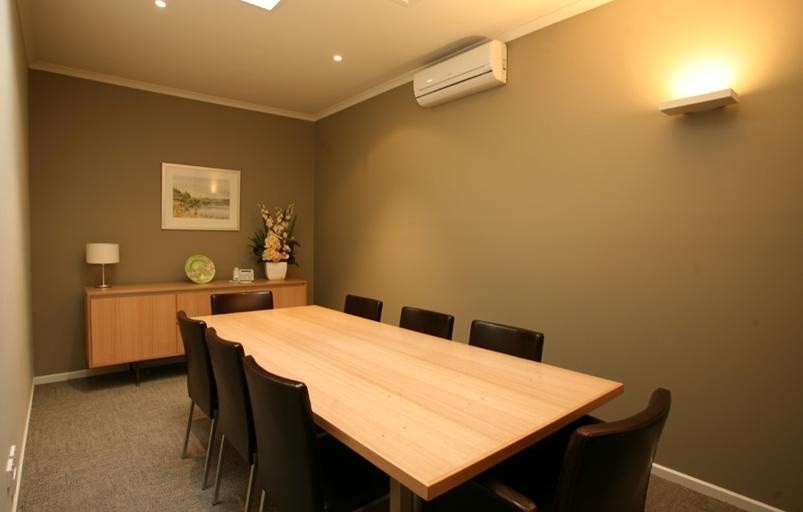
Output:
[190,303,627,512]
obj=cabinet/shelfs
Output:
[81,276,313,388]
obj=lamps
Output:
[658,88,741,116]
[85,242,120,287]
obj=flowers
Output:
[245,198,303,270]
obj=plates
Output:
[184,254,215,284]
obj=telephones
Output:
[233,267,254,282]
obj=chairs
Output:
[343,293,385,321]
[502,389,674,511]
[469,318,544,365]
[175,310,217,491]
[202,327,258,512]
[399,305,456,341]
[240,356,378,512]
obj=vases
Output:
[265,261,287,280]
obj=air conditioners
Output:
[412,38,508,109]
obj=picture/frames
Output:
[160,159,241,232]
